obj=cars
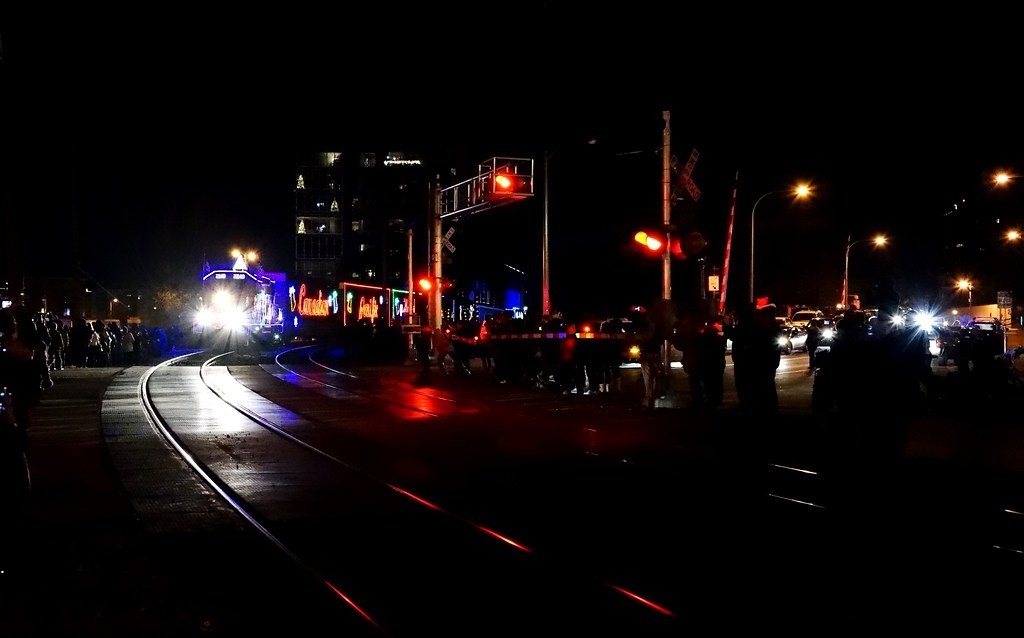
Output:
[722,307,838,356]
[891,308,1009,359]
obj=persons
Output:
[803,322,819,371]
[994,318,1002,326]
[672,316,725,409]
[724,301,779,415]
[638,333,661,408]
[543,314,625,398]
[410,318,473,376]
[967,318,977,327]
[0,299,177,494]
[952,320,960,325]
[830,310,879,352]
[479,314,532,386]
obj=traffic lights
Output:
[489,168,523,197]
[633,228,667,257]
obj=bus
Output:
[187,246,429,347]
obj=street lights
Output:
[954,278,972,315]
[42,298,47,320]
[109,298,118,319]
[844,232,887,312]
[749,181,816,305]
